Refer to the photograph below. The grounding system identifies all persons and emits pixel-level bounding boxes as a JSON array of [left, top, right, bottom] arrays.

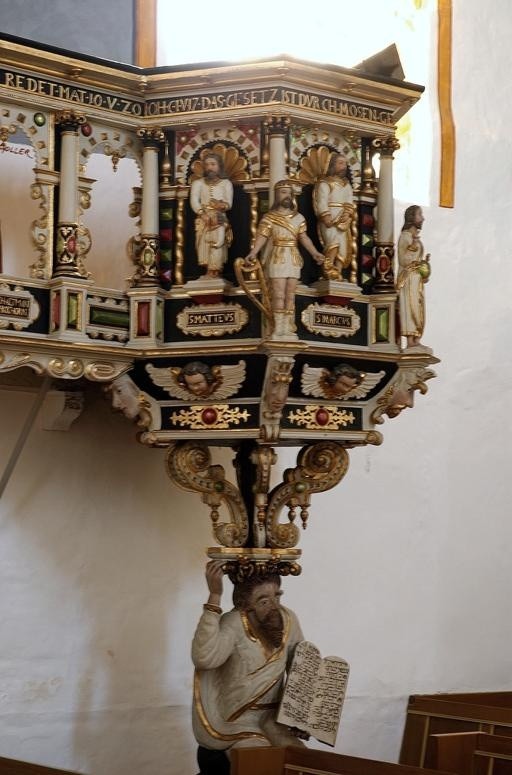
[[326, 366, 367, 396], [189, 153, 233, 274], [104, 374, 145, 421], [314, 155, 360, 278], [178, 361, 221, 396], [191, 557, 321, 753], [386, 380, 429, 419], [244, 181, 325, 340], [396, 205, 431, 349]]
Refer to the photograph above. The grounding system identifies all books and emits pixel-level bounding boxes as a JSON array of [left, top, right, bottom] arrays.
[[276, 641, 349, 747]]
